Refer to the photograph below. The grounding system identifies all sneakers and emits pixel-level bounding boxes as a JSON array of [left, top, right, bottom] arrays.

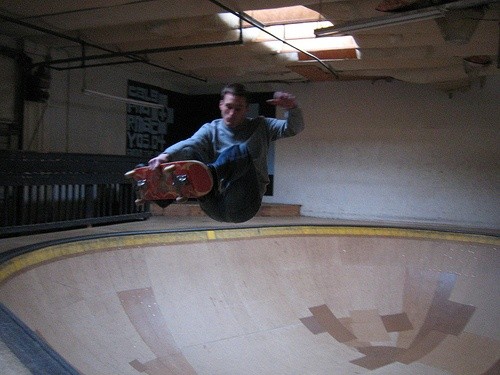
[[136, 163, 168, 208], [182, 147, 212, 172]]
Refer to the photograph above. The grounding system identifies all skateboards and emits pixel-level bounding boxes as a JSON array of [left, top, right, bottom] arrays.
[[125, 160, 212, 205]]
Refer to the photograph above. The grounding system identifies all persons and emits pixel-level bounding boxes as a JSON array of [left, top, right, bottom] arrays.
[[136, 82, 305, 223]]
